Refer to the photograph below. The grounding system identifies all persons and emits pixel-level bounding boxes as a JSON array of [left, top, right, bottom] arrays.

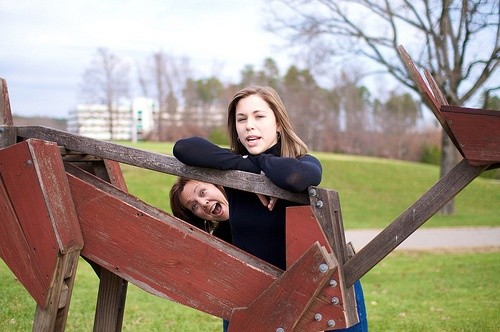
[[172, 86, 368, 332], [169, 176, 232, 244]]
